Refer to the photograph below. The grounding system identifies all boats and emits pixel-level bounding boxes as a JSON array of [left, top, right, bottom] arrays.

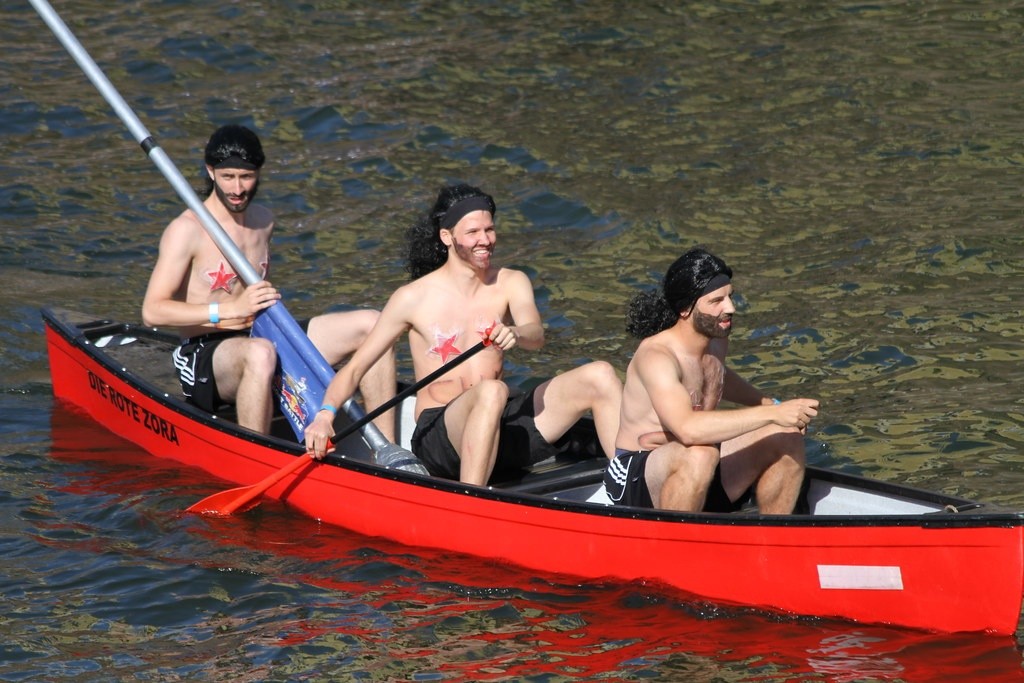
[[45, 309, 1022, 638]]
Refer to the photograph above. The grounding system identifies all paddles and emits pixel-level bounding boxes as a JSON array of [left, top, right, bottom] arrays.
[[187, 322, 503, 517]]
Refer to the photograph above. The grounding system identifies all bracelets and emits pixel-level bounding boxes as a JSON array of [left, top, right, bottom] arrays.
[[209, 302, 218, 324], [318, 405, 336, 416], [772, 398, 781, 405]]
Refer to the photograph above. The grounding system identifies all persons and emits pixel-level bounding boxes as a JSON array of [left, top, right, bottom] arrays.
[[603, 247, 820, 515], [141, 125, 397, 442], [305, 185, 624, 485]]
